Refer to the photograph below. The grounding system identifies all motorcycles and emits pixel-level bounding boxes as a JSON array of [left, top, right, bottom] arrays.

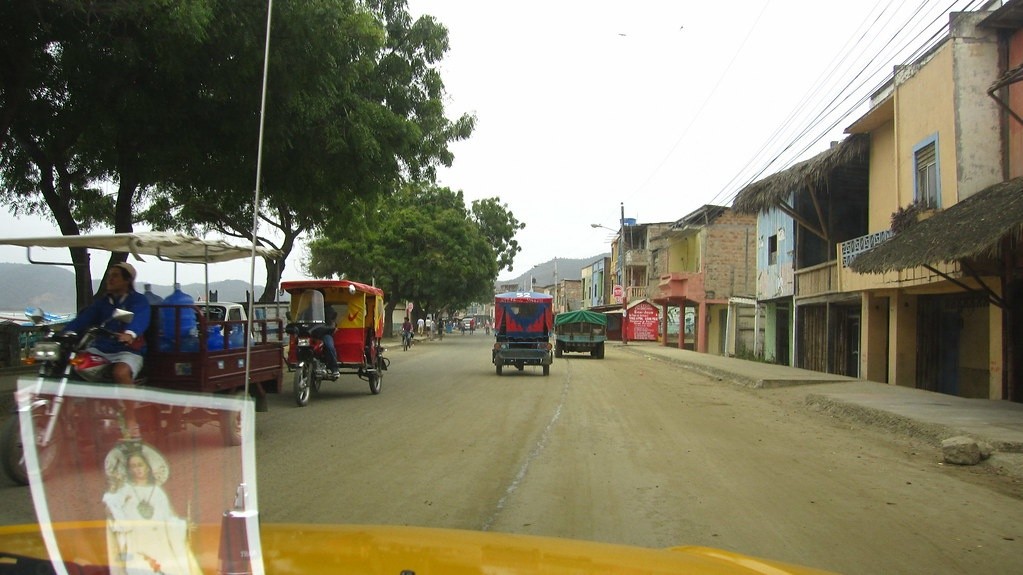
[[490, 290, 555, 377], [280, 280, 389, 408], [2, 232, 285, 483]]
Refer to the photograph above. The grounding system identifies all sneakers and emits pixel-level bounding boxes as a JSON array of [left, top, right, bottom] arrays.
[[332, 371, 341, 378]]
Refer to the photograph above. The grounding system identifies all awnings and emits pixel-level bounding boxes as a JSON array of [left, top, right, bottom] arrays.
[[851, 176, 1021, 318], [729, 131, 867, 243]]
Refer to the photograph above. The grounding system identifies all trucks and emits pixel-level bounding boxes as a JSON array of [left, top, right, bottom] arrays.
[[555, 310, 607, 359]]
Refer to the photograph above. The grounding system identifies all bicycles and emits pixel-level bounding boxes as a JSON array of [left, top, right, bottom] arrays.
[[401, 331, 416, 351]]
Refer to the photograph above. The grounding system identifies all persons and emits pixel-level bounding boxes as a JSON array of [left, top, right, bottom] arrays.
[[417, 315, 496, 342], [400, 316, 413, 348], [296, 288, 341, 378], [55, 261, 154, 428]]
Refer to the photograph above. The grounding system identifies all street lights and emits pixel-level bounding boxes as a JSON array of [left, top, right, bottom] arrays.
[[591, 202, 628, 345]]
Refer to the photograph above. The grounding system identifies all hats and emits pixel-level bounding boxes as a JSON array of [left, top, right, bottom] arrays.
[[110, 261, 136, 281]]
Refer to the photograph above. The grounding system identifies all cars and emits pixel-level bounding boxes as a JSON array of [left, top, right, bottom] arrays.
[[444, 315, 491, 334]]
